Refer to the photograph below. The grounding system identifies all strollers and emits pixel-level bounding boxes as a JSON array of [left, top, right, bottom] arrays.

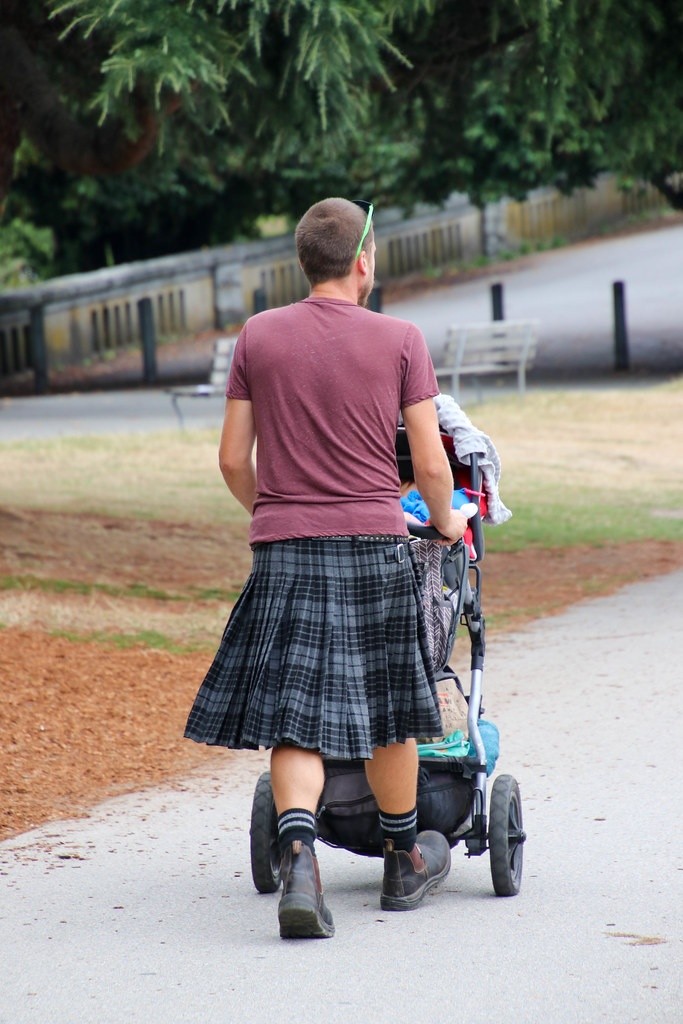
[[250, 394, 529, 897]]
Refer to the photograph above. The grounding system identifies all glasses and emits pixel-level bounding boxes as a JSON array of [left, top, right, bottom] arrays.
[[351, 200, 375, 259]]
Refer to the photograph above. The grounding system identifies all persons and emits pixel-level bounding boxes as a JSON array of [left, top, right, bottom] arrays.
[[395, 429, 472, 527], [184, 198, 467, 938]]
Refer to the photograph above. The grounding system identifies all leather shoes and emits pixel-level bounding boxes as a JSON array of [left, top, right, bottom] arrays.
[[278, 840, 335, 938], [380, 831, 451, 911]]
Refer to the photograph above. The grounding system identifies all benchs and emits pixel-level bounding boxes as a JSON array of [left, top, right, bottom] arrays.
[[164, 336, 236, 431], [434, 319, 538, 401]]
